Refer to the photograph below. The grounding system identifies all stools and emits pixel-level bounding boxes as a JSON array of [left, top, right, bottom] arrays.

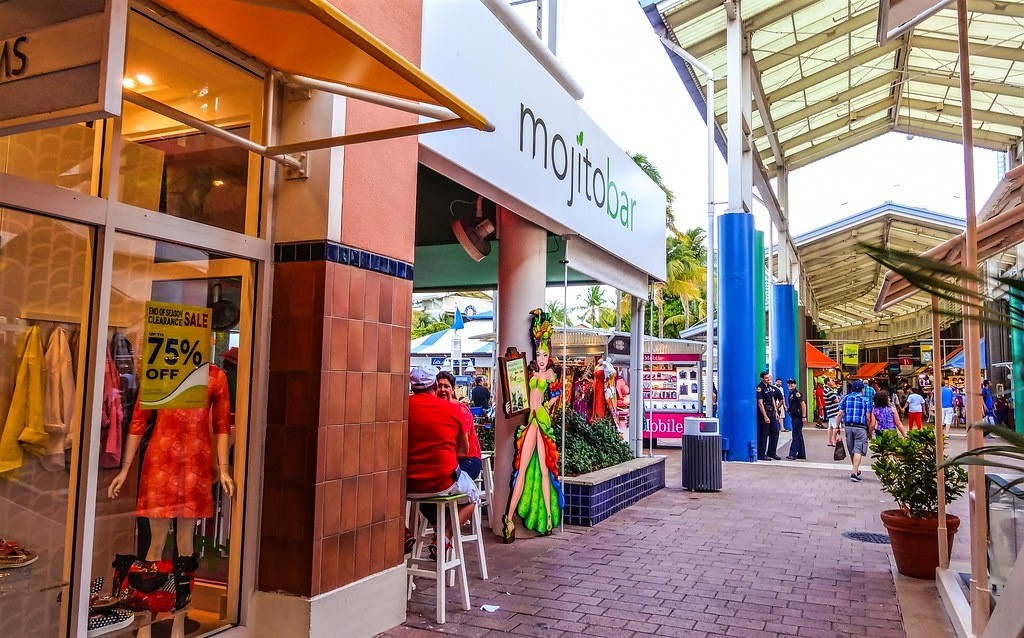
[[406, 449, 493, 624]]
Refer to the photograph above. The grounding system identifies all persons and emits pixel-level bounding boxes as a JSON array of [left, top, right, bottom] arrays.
[[403, 364, 492, 560], [758, 369, 1013, 484], [110, 338, 232, 638]]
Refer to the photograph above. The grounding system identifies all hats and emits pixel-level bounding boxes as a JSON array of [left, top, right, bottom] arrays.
[[851, 379, 866, 389], [409, 363, 440, 390], [218, 346, 238, 366], [785, 378, 796, 383]]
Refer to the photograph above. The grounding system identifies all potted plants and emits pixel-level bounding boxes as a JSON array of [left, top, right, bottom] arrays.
[[868, 425, 968, 580]]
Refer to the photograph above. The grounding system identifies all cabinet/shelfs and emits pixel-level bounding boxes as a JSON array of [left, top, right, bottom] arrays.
[[643, 369, 677, 399]]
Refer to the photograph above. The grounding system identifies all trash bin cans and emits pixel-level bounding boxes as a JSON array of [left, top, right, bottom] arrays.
[[681, 415, 723, 491]]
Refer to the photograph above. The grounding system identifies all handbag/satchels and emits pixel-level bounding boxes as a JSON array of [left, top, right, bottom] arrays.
[[833, 435, 846, 461]]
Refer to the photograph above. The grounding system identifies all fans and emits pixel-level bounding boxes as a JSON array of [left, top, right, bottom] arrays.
[[452, 194, 495, 263]]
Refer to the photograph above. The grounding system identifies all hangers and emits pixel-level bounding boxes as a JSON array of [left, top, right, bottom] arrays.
[[580, 360, 594, 382]]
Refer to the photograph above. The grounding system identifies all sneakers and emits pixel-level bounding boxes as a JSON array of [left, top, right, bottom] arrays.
[[0, 538, 40, 570]]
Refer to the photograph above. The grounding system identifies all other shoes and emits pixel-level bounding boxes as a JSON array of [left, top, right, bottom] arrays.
[[850, 470, 862, 482], [405, 526, 416, 551], [786, 455, 797, 460], [781, 429, 789, 432], [827, 443, 836, 447], [946, 435, 952, 440], [985, 434, 995, 440], [816, 422, 827, 429], [767, 454, 781, 460], [796, 454, 806, 459], [58, 576, 135, 638], [757, 454, 773, 460], [427, 539, 454, 563]]
